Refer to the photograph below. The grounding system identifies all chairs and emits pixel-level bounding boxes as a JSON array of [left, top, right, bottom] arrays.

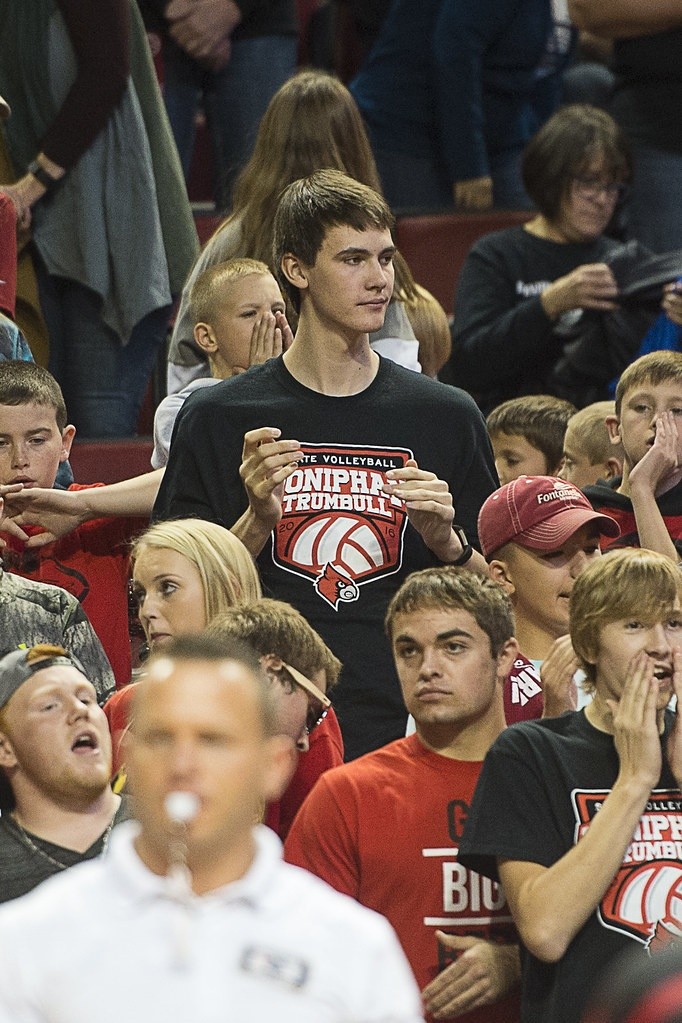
[[60, 439, 157, 553], [397, 209, 534, 310]]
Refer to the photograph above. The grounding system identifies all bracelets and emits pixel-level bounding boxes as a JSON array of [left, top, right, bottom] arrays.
[[28, 161, 63, 194]]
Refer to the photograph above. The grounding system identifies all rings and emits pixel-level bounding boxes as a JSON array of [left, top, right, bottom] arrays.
[[202, 46, 211, 56]]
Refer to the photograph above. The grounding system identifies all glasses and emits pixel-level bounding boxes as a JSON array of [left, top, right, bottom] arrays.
[[571, 176, 624, 201], [281, 663, 331, 731]]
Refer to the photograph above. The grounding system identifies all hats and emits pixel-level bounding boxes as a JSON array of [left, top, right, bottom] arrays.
[[477, 474, 622, 558], [0, 647, 86, 708]]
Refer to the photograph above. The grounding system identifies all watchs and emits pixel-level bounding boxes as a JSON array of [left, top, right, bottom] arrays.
[[430, 525, 472, 567]]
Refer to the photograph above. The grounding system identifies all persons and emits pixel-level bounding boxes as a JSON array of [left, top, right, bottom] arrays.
[[0, 360, 165, 708], [0, 643, 131, 905], [0, 311, 74, 489], [149, 75, 454, 471], [136, 0, 682, 272], [148, 167, 501, 753], [1, 0, 197, 434], [0, 631, 426, 1023], [285, 348, 682, 1022], [96, 516, 343, 838], [454, 100, 681, 395]]
